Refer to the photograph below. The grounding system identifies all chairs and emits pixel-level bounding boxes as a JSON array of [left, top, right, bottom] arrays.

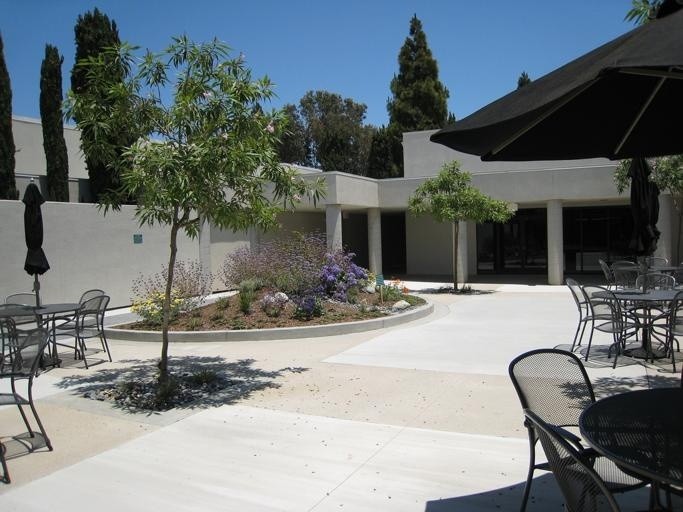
[[1, 289, 112, 486], [565, 255, 682, 381], [522, 409, 666, 512], [509, 351, 663, 512]]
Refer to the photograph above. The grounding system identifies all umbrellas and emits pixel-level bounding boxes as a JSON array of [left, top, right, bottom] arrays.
[[622, 159, 668, 360], [16, 176, 64, 370], [646, 178, 662, 255], [429, 2, 683, 162]]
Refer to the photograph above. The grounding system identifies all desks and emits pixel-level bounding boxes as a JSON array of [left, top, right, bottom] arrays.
[[579, 380, 681, 512]]
